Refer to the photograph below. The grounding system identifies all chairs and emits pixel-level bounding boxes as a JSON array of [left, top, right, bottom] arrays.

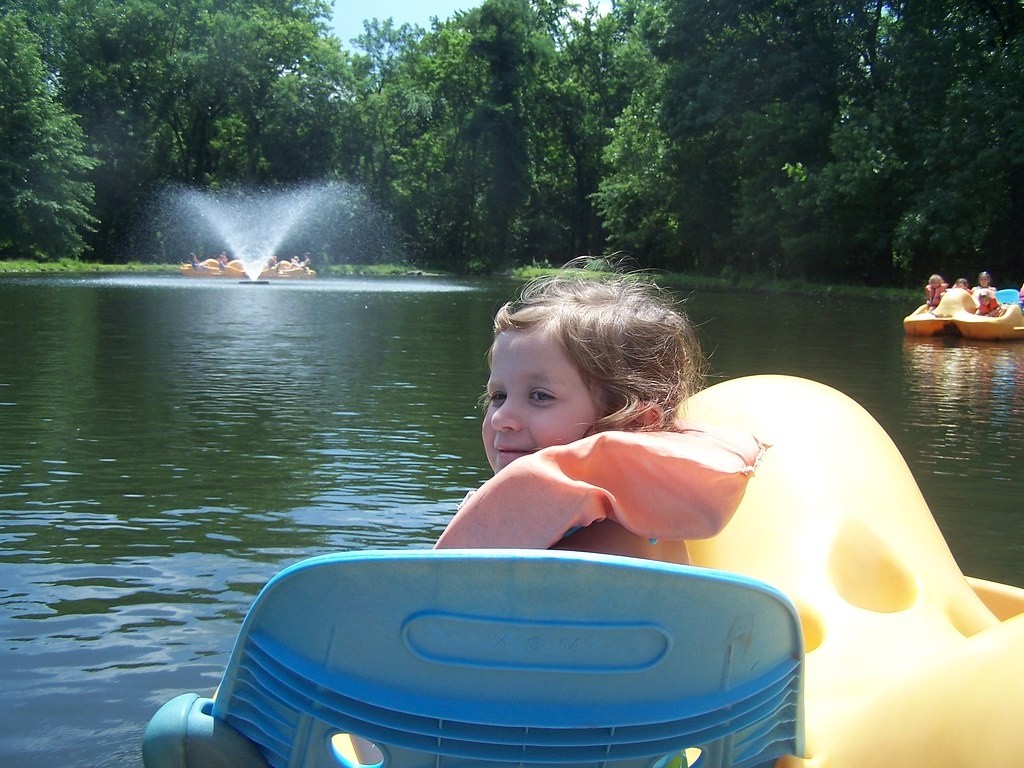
[[995, 289, 1019, 305], [138, 551, 802, 768]]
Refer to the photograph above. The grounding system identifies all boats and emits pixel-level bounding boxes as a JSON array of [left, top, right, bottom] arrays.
[[180, 258, 316, 279], [903, 288, 1024, 341]]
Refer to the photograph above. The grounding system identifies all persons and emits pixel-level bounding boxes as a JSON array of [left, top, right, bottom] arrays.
[[189, 251, 312, 271], [434, 256, 771, 565], [951, 278, 972, 295], [924, 274, 949, 310], [969, 271, 1024, 317]]
